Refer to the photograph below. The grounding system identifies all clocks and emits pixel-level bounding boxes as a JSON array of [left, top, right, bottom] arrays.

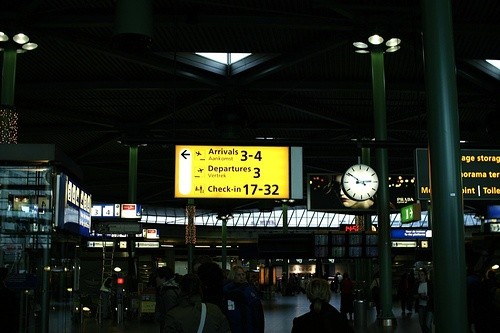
[[340, 156, 379, 201]]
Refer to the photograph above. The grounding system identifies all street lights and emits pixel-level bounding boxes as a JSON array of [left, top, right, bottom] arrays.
[[274, 199, 295, 278], [0, 30, 38, 124], [116, 140, 149, 254], [351, 32, 402, 328], [217, 213, 231, 272], [352, 138, 376, 231]]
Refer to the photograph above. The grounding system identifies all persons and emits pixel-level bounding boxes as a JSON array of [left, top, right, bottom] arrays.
[[341, 273, 354, 320], [281, 273, 300, 296], [325, 175, 374, 209], [99, 274, 117, 319], [292, 278, 352, 333], [155, 262, 264, 333], [0, 268, 8, 289], [397, 269, 432, 333]]
